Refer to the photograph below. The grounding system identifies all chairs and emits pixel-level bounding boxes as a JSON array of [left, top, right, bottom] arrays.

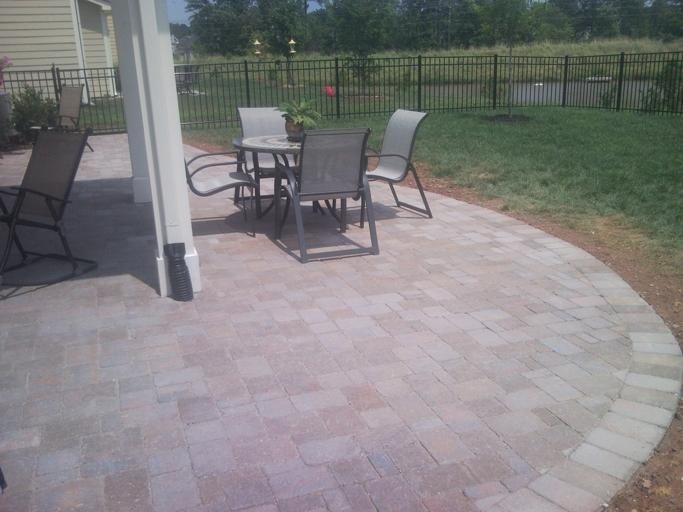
[[29, 83, 94, 152], [273, 126, 379, 263], [185, 150, 260, 229], [237, 107, 286, 182], [0, 125, 98, 286], [364, 107, 432, 218]]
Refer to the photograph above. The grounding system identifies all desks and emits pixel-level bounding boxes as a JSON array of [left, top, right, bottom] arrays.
[[232, 134, 349, 229]]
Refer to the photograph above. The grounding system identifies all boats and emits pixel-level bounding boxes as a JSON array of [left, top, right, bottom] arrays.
[[584, 73, 612, 82], [528, 81, 543, 87]]
